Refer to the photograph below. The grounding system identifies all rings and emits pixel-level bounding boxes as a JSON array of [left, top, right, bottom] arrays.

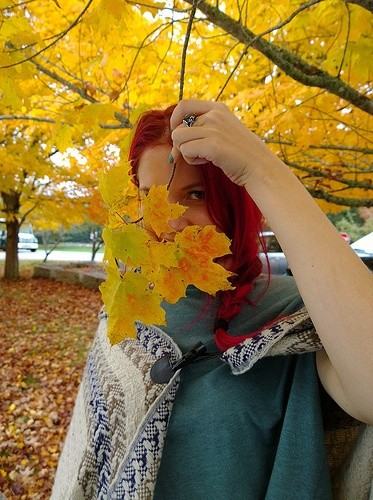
[[181, 113, 196, 129]]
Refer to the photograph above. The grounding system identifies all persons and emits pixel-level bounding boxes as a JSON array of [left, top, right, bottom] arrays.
[[48, 98, 373, 500]]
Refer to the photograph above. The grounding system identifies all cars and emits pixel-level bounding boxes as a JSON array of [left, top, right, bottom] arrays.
[[258, 228, 290, 277], [0, 218, 39, 253], [350, 231, 373, 273]]
